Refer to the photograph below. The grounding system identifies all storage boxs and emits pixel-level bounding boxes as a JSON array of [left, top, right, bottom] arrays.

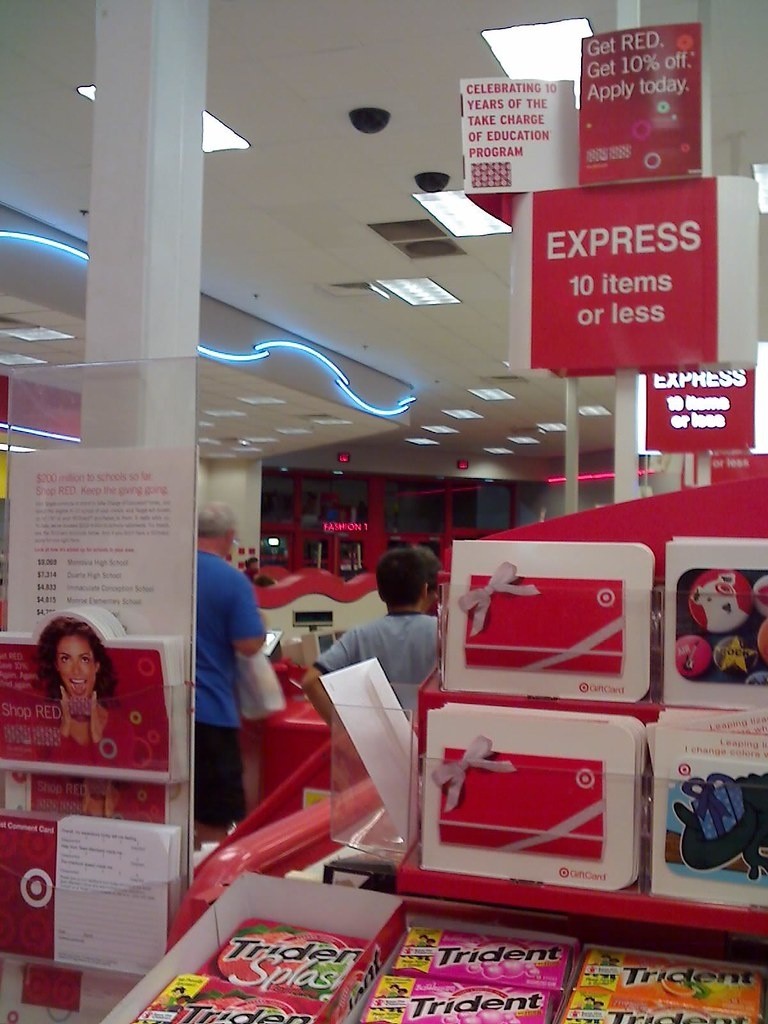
[[93, 872, 407, 1024], [555, 943, 768, 1024], [344, 916, 578, 1024]]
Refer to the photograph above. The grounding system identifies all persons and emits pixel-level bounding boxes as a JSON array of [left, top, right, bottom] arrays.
[[243, 557, 259, 583], [193, 500, 266, 850], [301, 547, 439, 731], [34, 616, 118, 744]]
